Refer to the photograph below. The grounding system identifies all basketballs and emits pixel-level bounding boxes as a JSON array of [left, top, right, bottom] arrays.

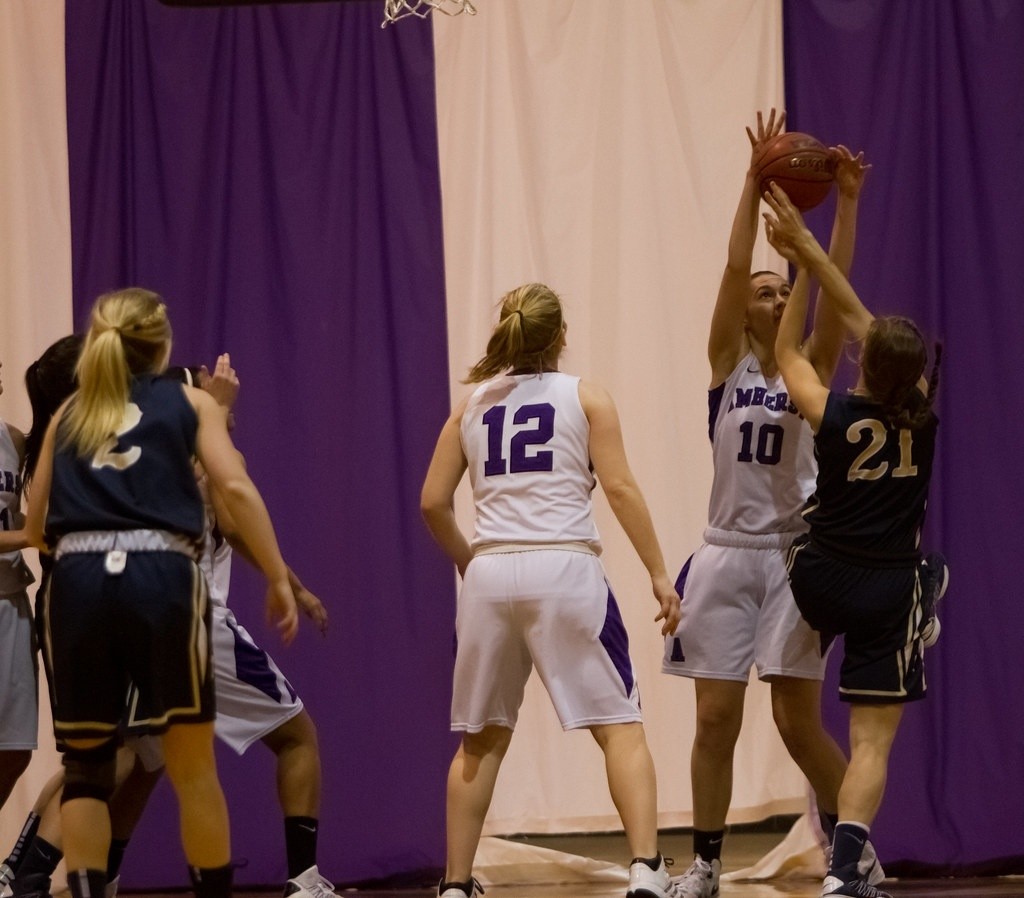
[[754, 129, 834, 213]]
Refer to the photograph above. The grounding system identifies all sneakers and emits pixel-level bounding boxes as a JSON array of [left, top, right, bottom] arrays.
[[675, 856, 720, 897], [625, 851, 687, 898], [822, 869, 895, 898], [436, 875, 485, 898], [821, 845, 886, 887], [8, 874, 52, 898], [284, 864, 343, 898], [919, 561, 952, 647]]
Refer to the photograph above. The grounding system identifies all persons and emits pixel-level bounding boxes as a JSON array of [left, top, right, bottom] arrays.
[[666, 109, 886, 897], [418, 280, 685, 896], [752, 177, 950, 898], [1, 285, 346, 897]]
